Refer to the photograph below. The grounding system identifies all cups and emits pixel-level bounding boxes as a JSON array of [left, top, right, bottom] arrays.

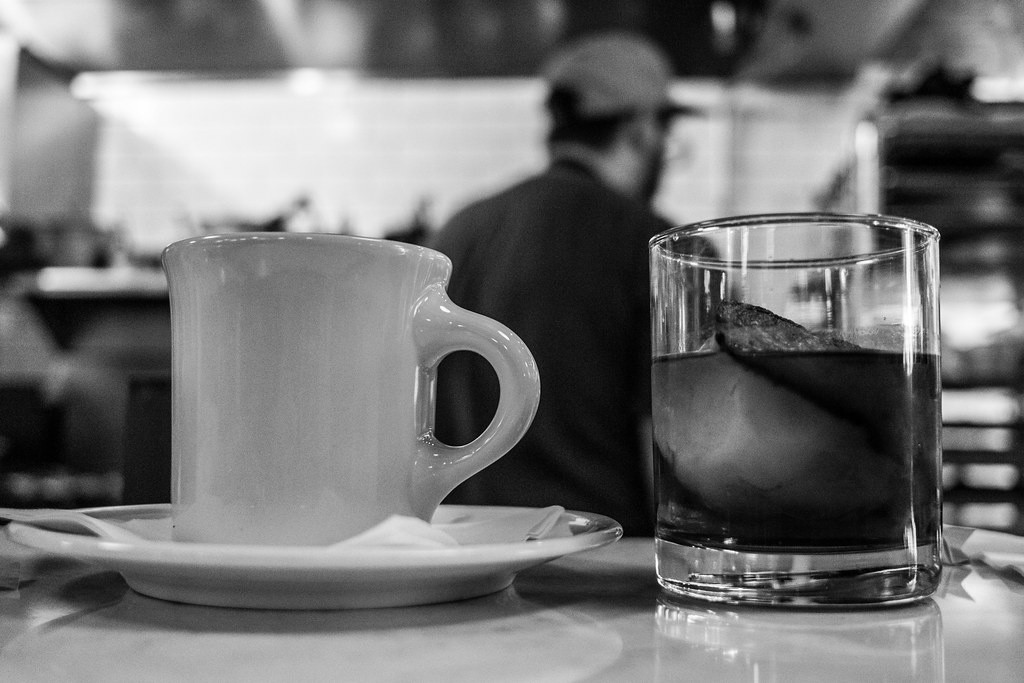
[[160, 232, 541, 546], [648, 212, 945, 605]]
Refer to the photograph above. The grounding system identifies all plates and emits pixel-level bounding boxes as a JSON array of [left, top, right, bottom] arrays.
[[3, 503, 623, 611]]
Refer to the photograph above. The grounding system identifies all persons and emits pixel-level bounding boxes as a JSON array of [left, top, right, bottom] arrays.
[[428, 30, 734, 540]]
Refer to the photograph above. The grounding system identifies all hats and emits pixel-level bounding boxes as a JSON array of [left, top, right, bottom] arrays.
[[540, 30, 708, 128]]
